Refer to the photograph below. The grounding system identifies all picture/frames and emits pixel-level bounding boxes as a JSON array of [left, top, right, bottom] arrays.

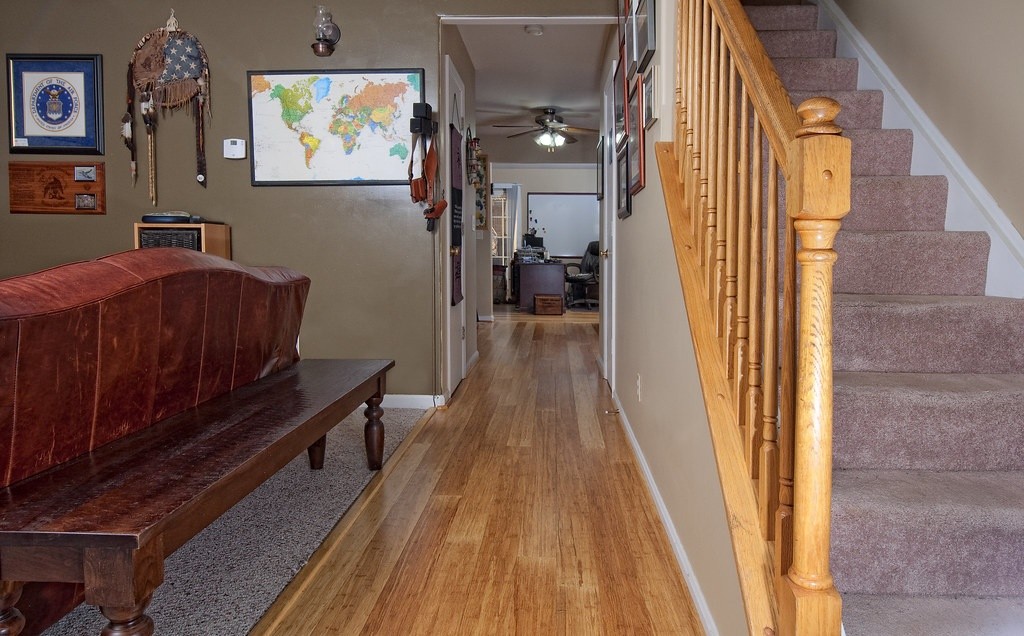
[[5, 52, 107, 157], [246, 67, 426, 188], [595, 0, 661, 220]]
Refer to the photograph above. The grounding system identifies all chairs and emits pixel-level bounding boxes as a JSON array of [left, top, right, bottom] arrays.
[[563, 241, 599, 310]]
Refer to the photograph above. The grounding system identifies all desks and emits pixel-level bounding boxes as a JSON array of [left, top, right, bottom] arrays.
[[0, 358, 398, 636], [511, 258, 567, 314]]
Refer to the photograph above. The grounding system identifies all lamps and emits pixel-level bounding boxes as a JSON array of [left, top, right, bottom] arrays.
[[310, 4, 340, 58], [533, 129, 568, 153]]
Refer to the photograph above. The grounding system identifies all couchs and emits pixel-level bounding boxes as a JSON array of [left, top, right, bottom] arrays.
[[0, 247, 313, 636]]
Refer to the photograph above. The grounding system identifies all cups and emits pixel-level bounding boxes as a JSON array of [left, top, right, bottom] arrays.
[[543, 250, 549, 262]]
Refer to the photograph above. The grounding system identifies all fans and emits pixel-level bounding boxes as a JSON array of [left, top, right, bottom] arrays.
[[491, 108, 600, 144]]
